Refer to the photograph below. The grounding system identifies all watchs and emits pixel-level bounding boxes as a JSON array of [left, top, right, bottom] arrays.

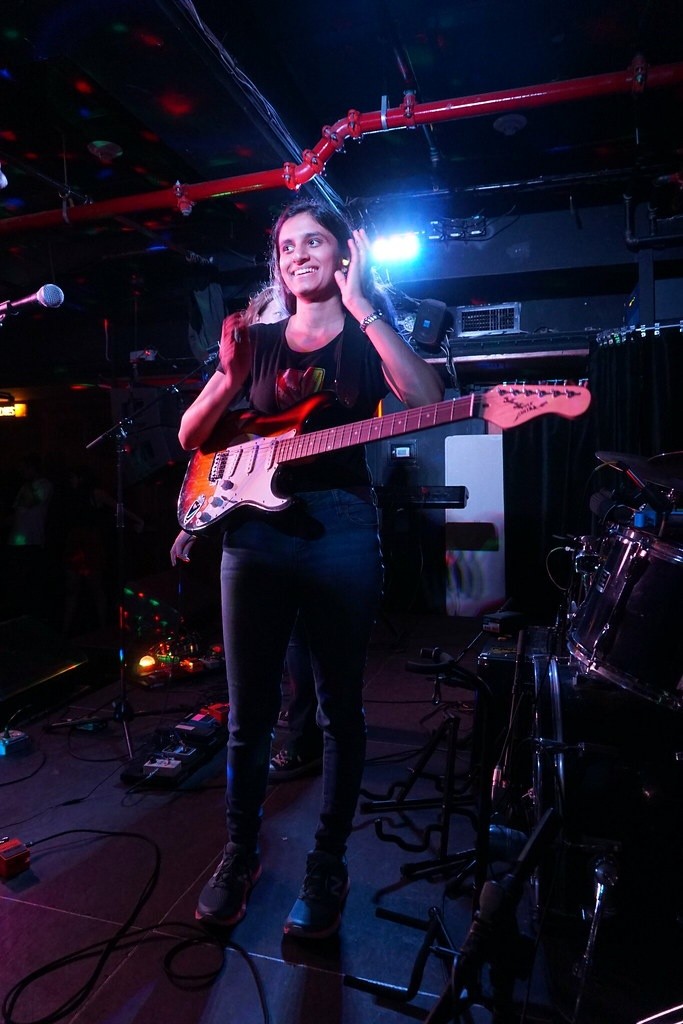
[[360, 309, 387, 333]]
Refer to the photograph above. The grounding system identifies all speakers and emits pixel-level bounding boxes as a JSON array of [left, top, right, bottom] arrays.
[[467, 625, 569, 793], [414, 299, 448, 351]]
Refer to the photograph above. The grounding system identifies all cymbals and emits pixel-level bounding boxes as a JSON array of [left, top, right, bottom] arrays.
[[590, 449, 682, 493]]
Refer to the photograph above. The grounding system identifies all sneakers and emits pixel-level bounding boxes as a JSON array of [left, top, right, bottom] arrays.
[[283, 851, 352, 940], [277, 711, 290, 727], [267, 746, 314, 780], [194, 843, 264, 927]]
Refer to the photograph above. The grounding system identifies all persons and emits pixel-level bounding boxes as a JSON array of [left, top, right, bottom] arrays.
[[170, 200, 444, 939]]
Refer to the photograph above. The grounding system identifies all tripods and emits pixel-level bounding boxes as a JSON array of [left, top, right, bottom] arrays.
[[39, 355, 216, 773]]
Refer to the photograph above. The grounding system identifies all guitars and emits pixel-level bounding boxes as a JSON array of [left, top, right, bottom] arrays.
[[173, 380, 595, 537]]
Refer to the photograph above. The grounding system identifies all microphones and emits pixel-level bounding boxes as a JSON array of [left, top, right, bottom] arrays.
[[616, 459, 673, 516], [0, 284, 64, 316]]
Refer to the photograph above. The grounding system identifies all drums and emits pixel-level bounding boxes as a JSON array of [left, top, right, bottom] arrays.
[[565, 516, 683, 711]]
